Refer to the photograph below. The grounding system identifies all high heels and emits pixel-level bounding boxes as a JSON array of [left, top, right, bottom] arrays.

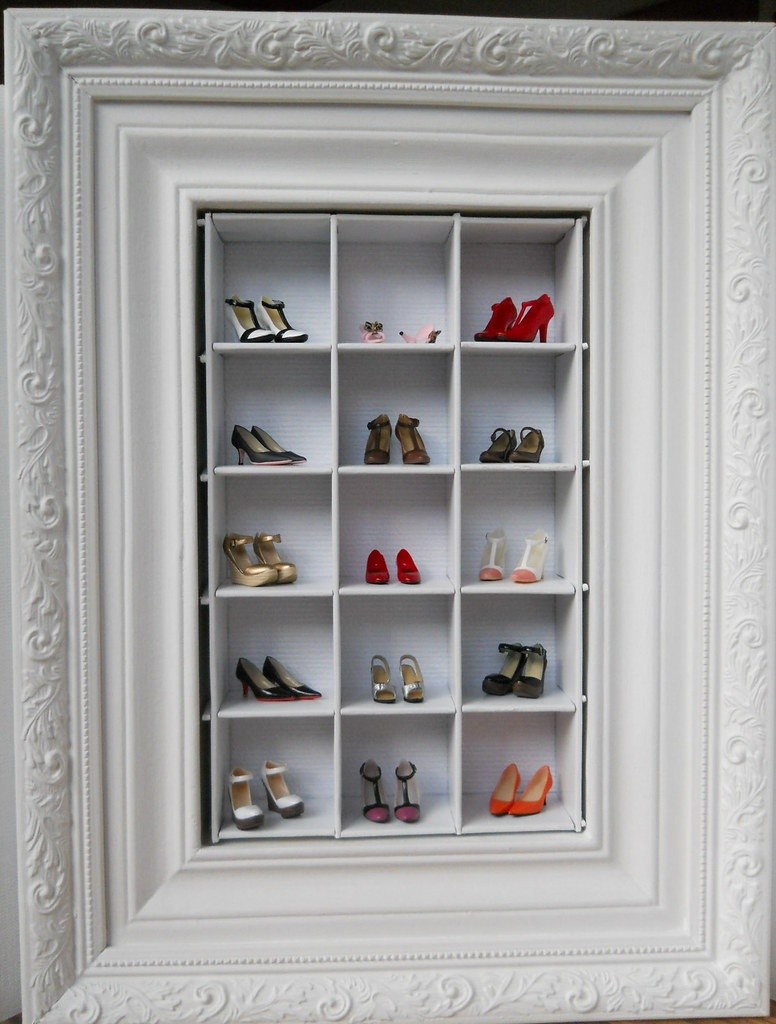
[[490, 763, 521, 816], [223, 530, 279, 587], [479, 527, 507, 581], [479, 427, 517, 463], [399, 655, 424, 703], [359, 757, 389, 823], [394, 758, 420, 821], [370, 654, 396, 703], [509, 528, 552, 583], [224, 294, 308, 343], [232, 424, 307, 465], [508, 765, 553, 816], [365, 549, 390, 584], [364, 414, 392, 464], [253, 530, 297, 584], [359, 320, 385, 342], [396, 549, 421, 584], [399, 323, 441, 344], [228, 767, 264, 830], [482, 642, 547, 699], [510, 427, 544, 463], [395, 413, 431, 464], [474, 294, 554, 343], [235, 655, 322, 701], [261, 760, 304, 819]]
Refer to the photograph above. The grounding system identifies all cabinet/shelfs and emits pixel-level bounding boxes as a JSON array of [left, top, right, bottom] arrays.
[[196, 214, 586, 844]]
